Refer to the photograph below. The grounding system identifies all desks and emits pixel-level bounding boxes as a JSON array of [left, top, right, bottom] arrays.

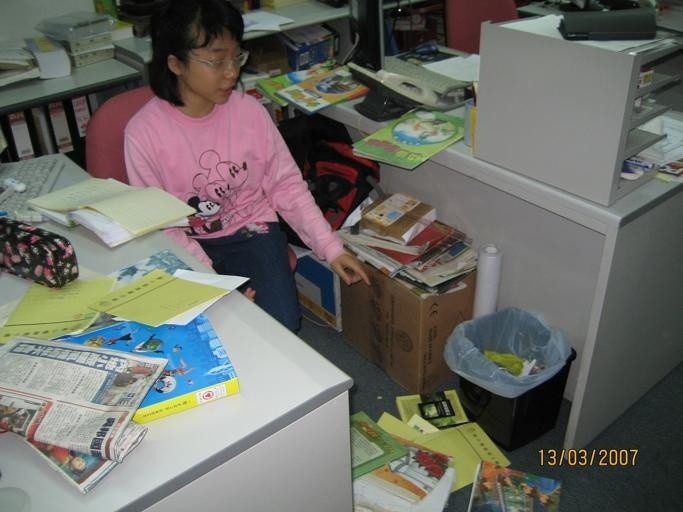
[[320, 16, 683, 459], [0, 154, 355, 512]]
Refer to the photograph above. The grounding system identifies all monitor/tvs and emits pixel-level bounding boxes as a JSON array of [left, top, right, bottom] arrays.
[[341, 0, 385, 70]]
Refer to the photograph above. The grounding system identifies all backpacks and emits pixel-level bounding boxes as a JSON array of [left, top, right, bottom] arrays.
[[277, 117, 379, 250]]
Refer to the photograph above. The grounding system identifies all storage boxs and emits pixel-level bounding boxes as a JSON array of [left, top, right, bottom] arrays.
[[340, 263, 476, 394]]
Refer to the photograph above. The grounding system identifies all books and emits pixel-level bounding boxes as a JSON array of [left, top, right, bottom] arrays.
[[335, 191, 479, 297], [0, 37, 71, 85], [348, 106, 468, 172], [28, 177, 190, 246], [274, 66, 370, 113], [48, 312, 240, 424], [255, 65, 331, 107], [25, 177, 197, 236], [349, 388, 562, 511]]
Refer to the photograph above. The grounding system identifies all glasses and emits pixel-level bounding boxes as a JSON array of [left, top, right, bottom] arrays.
[[187, 47, 250, 74]]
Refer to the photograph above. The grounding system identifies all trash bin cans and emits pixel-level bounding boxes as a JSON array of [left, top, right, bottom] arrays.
[[458, 307, 576, 451]]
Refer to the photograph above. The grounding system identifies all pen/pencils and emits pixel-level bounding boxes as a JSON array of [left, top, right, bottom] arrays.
[[471, 81, 477, 108]]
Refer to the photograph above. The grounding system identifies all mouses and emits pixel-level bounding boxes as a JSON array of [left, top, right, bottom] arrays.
[[412, 40, 438, 55]]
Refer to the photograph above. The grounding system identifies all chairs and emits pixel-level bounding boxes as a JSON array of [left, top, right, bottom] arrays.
[[85, 87, 298, 289]]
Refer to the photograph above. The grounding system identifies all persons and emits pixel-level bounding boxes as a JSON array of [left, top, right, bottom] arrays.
[[25, 438, 88, 476], [121, 2, 370, 333]]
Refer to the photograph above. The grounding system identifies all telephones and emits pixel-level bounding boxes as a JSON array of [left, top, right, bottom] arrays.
[[345, 61, 438, 122]]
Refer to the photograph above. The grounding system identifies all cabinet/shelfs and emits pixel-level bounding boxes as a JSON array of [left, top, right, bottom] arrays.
[[0, 55, 141, 170], [112, 3, 351, 171]]
